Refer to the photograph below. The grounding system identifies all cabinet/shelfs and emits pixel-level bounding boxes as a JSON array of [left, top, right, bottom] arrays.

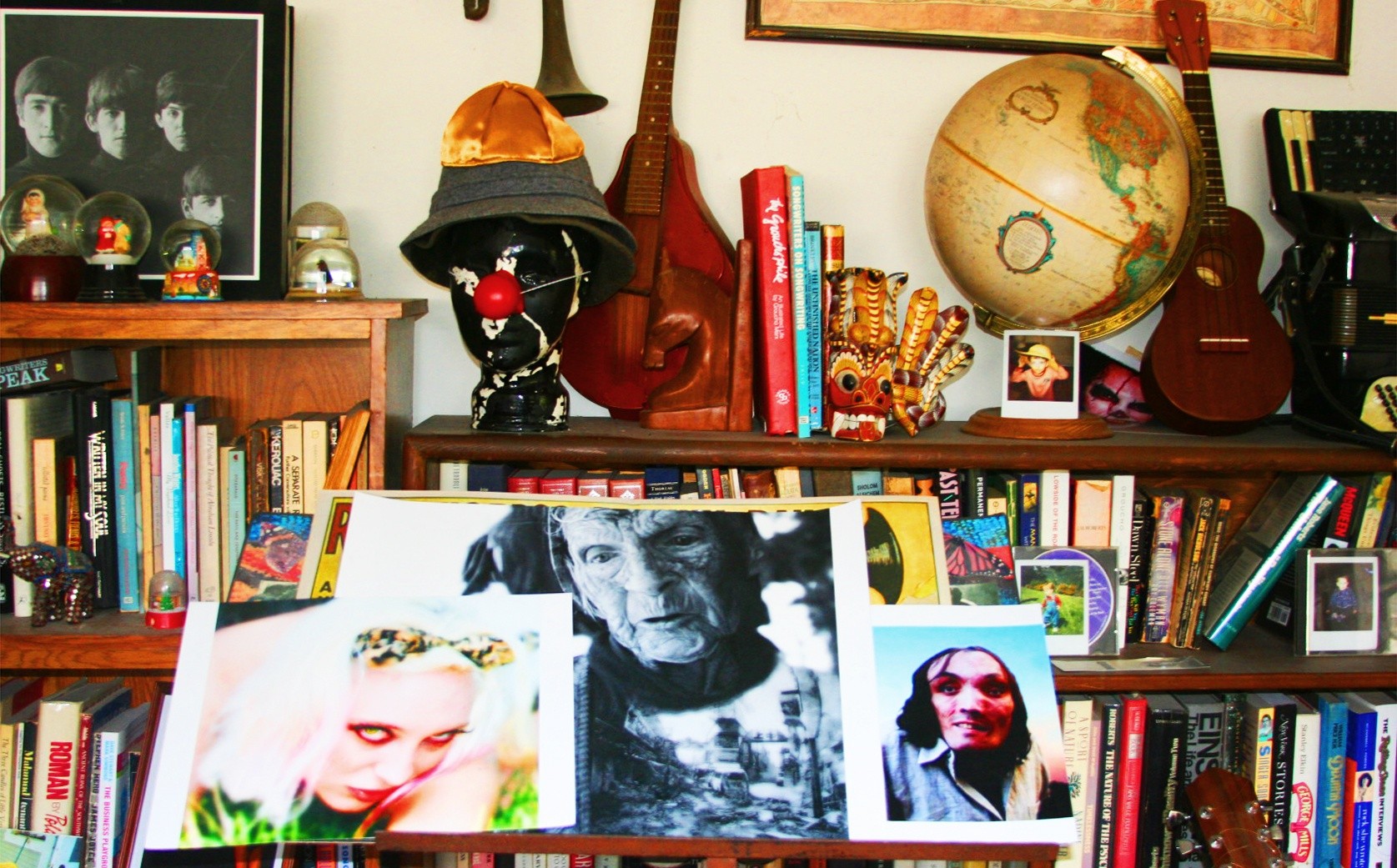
[[1, 292, 429, 867], [409, 415, 1396, 867]]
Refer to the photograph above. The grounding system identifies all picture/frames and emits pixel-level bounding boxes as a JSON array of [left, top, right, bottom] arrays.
[[743, 0, 1354, 77], [1294, 548, 1396, 657]]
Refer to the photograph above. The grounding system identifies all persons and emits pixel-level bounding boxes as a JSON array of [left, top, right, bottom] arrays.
[[178, 154, 250, 275], [146, 67, 231, 178], [444, 209, 595, 433], [80, 61, 175, 224], [7, 51, 110, 195]]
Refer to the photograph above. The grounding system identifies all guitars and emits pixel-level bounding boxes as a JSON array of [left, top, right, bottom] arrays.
[[556, 0, 739, 415], [1139, 1, 1295, 429], [1164, 764, 1291, 868]]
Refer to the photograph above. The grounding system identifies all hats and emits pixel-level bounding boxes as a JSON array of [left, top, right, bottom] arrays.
[[410, 211, 636, 310], [400, 81, 640, 289]]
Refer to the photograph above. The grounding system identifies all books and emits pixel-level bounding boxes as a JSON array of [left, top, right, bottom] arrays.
[[0, 344, 1394, 868]]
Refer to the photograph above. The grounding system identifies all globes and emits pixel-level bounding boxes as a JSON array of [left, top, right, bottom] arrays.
[[923, 43, 1208, 442]]
